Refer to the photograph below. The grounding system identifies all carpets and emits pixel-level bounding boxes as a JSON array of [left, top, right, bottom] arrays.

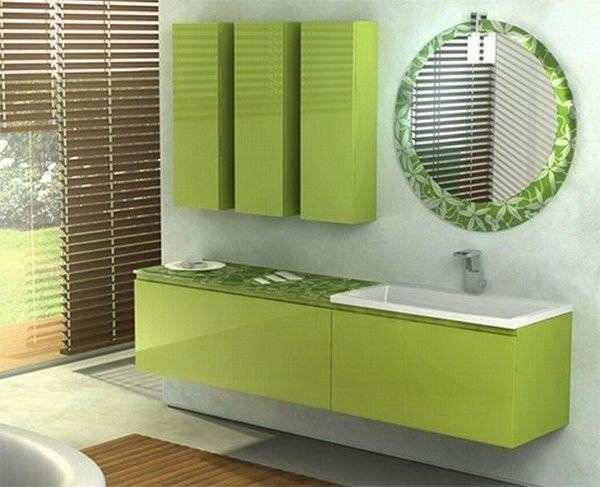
[[78, 433, 344, 487]]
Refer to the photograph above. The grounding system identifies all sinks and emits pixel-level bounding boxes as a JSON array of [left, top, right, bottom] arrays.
[[331, 281, 572, 327], [0, 425, 107, 487]]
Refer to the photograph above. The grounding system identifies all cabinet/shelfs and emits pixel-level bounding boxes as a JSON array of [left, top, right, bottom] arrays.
[[133, 279, 574, 450], [233, 23, 300, 218], [172, 22, 235, 212], [299, 21, 378, 224]]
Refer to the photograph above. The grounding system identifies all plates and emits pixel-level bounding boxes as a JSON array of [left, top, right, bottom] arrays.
[[165, 260, 226, 273]]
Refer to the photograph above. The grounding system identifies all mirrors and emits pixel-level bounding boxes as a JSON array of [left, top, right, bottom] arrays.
[[392, 19, 577, 232]]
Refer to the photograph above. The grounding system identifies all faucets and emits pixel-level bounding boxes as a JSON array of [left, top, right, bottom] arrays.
[[452, 249, 488, 295]]
